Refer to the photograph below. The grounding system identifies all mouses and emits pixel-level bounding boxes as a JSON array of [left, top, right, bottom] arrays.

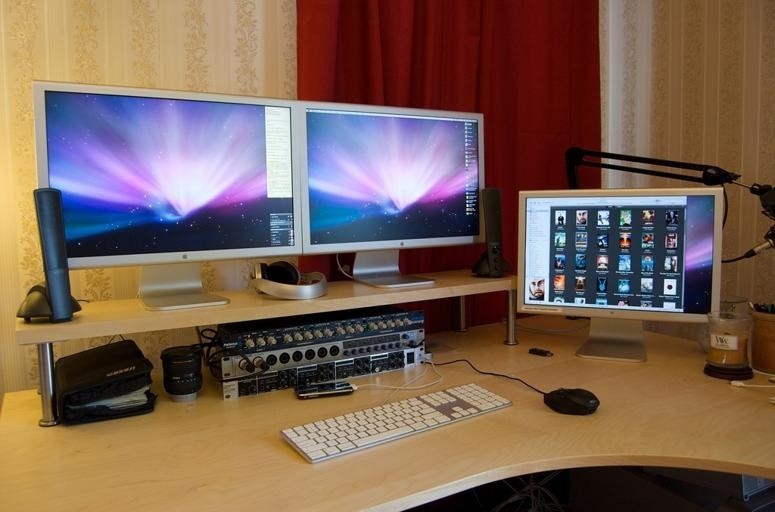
[[544, 389, 601, 415]]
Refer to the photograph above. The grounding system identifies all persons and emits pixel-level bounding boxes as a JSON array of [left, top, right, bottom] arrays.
[[576, 210, 587, 225], [554, 213, 565, 226], [528, 279, 544, 298]]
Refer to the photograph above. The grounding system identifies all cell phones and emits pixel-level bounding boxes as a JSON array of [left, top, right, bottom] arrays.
[[295, 382, 354, 398]]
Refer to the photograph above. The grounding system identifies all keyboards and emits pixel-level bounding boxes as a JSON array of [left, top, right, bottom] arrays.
[[280, 381, 511, 466]]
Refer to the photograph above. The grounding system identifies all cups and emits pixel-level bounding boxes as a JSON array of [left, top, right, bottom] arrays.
[[160, 345, 204, 394], [705, 310, 754, 370]]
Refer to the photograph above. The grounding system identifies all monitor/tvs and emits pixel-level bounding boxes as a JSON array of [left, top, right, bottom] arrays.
[[297, 99, 487, 290], [32, 79, 297, 312], [516, 188, 721, 364]]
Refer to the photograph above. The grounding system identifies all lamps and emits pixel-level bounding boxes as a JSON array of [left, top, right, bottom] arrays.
[[565, 146, 774, 262]]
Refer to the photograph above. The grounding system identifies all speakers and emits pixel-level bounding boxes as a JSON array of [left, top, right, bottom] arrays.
[[482, 189, 503, 276], [34, 187, 71, 324]]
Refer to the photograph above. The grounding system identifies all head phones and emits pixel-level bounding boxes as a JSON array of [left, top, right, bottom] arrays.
[[249, 261, 329, 299]]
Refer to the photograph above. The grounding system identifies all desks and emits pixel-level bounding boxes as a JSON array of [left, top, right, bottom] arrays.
[[2, 314, 775, 510]]
